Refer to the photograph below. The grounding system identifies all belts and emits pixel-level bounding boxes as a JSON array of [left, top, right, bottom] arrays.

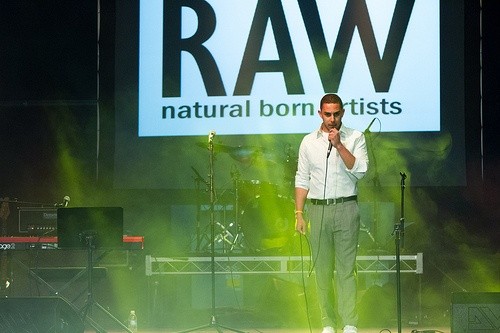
[[311, 196, 356, 205]]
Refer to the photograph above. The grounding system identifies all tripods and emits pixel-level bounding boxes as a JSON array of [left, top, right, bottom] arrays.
[[57, 206, 133, 333], [176, 143, 248, 333]]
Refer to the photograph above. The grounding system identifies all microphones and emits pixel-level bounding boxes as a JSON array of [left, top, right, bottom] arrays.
[[61, 195, 70, 207], [364, 118, 376, 135], [327, 142, 333, 158], [209, 131, 216, 143]]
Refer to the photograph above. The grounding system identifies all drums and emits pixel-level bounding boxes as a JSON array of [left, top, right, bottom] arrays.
[[235, 179, 274, 214], [284, 156, 299, 188], [240, 194, 297, 252]]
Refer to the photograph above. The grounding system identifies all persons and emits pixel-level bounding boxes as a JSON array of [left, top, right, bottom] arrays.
[[294, 94, 369, 333]]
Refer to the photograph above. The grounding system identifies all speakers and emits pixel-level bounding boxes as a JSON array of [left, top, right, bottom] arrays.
[[0, 294, 85, 333], [450, 292, 500, 333]]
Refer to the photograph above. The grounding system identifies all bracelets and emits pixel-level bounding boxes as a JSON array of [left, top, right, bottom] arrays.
[[295, 211, 303, 214]]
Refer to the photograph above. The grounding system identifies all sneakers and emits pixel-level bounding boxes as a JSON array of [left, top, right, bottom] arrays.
[[343, 326, 357, 333], [323, 327, 334, 333]]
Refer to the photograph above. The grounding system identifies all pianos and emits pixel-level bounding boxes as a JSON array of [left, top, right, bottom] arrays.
[[0, 234, 143, 333]]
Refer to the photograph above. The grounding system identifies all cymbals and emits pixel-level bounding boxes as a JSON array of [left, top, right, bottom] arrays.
[[209, 186, 234, 190], [196, 142, 234, 152], [229, 146, 282, 165]]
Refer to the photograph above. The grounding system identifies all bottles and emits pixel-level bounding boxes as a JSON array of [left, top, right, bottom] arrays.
[[128, 310, 137, 333]]
